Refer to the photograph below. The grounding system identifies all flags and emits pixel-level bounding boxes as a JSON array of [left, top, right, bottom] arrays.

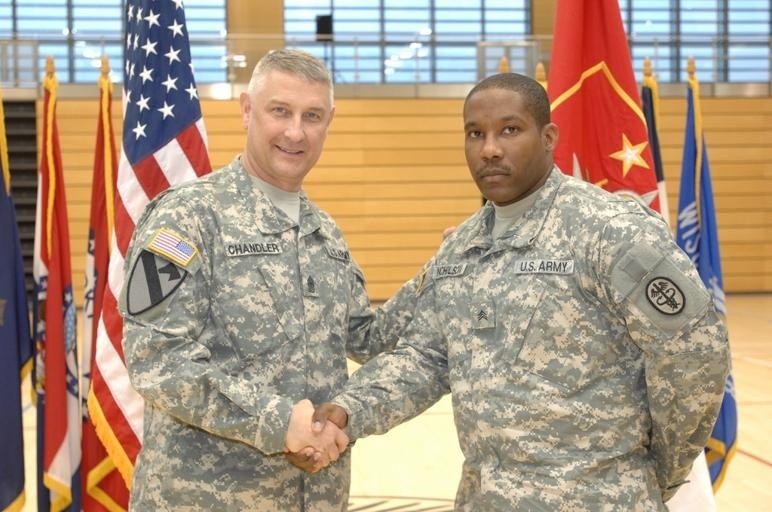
[[37, 53, 85, 511], [675, 56, 737, 493], [82, 1, 215, 510], [640, 58, 670, 229], [1, 90, 37, 512], [544, 1, 659, 216], [86, 57, 121, 399]]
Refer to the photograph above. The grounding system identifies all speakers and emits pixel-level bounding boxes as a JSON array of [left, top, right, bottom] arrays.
[[315, 14, 332, 41]]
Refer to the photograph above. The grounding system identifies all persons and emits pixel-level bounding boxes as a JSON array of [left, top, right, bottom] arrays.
[[286, 72, 733, 511], [116, 46, 459, 511]]
